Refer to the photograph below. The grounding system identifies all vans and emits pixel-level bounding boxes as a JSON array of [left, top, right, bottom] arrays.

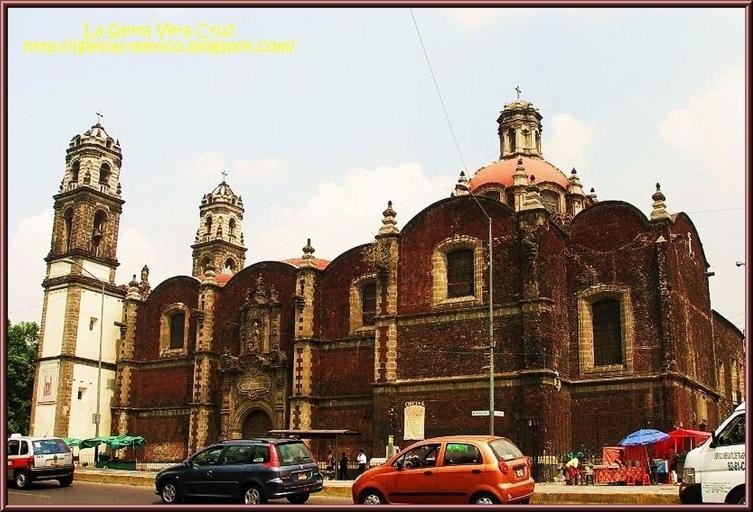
[[679, 401, 748, 504]]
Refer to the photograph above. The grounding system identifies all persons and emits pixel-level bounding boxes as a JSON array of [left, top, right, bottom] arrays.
[[565, 451, 584, 485], [396, 449, 419, 463], [326, 452, 351, 480], [356, 449, 367, 475]]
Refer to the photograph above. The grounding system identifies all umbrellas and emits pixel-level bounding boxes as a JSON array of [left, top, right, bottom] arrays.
[[617, 429, 671, 474]]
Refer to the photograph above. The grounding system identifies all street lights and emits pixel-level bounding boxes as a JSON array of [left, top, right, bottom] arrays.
[[455, 182, 494, 435], [59, 258, 106, 462]]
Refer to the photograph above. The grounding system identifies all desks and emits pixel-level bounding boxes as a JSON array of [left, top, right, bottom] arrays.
[[580, 466, 642, 486]]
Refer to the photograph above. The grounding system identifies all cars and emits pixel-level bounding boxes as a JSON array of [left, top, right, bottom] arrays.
[[352, 434, 535, 505], [155, 436, 324, 504], [7, 435, 75, 490]]
